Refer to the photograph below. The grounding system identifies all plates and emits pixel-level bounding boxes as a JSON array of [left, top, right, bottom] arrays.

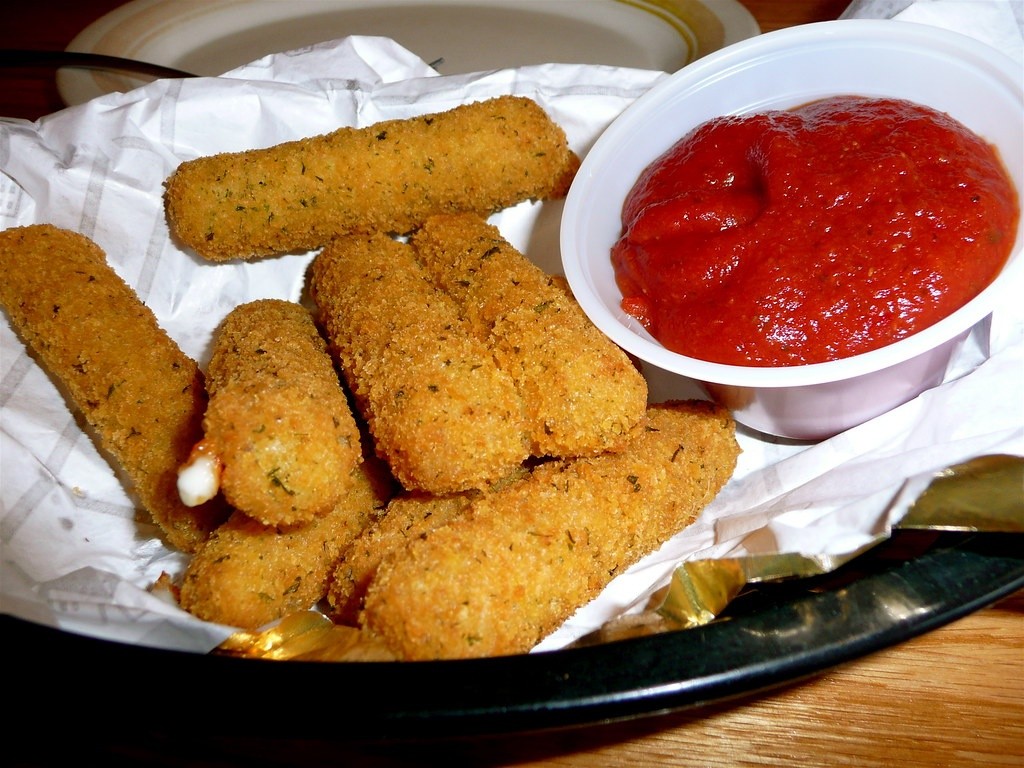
[[55, 0, 765, 109], [0, 535, 1024, 746]]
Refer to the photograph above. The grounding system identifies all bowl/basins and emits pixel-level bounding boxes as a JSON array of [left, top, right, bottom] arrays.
[[560, 17, 1024, 441]]
